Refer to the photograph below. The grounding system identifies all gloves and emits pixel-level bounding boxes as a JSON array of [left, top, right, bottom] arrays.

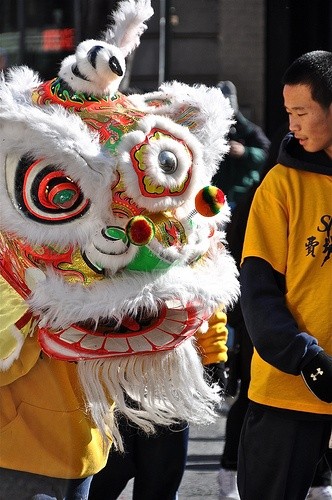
[[301, 348, 332, 407]]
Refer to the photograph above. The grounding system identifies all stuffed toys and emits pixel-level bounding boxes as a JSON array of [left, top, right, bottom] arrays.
[[2, 0, 245, 455]]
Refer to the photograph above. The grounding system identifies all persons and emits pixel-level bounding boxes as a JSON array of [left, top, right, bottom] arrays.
[[212, 317, 331, 500], [235, 48, 332, 500], [214, 79, 270, 402], [88, 303, 232, 500], [1, 324, 125, 500]]
